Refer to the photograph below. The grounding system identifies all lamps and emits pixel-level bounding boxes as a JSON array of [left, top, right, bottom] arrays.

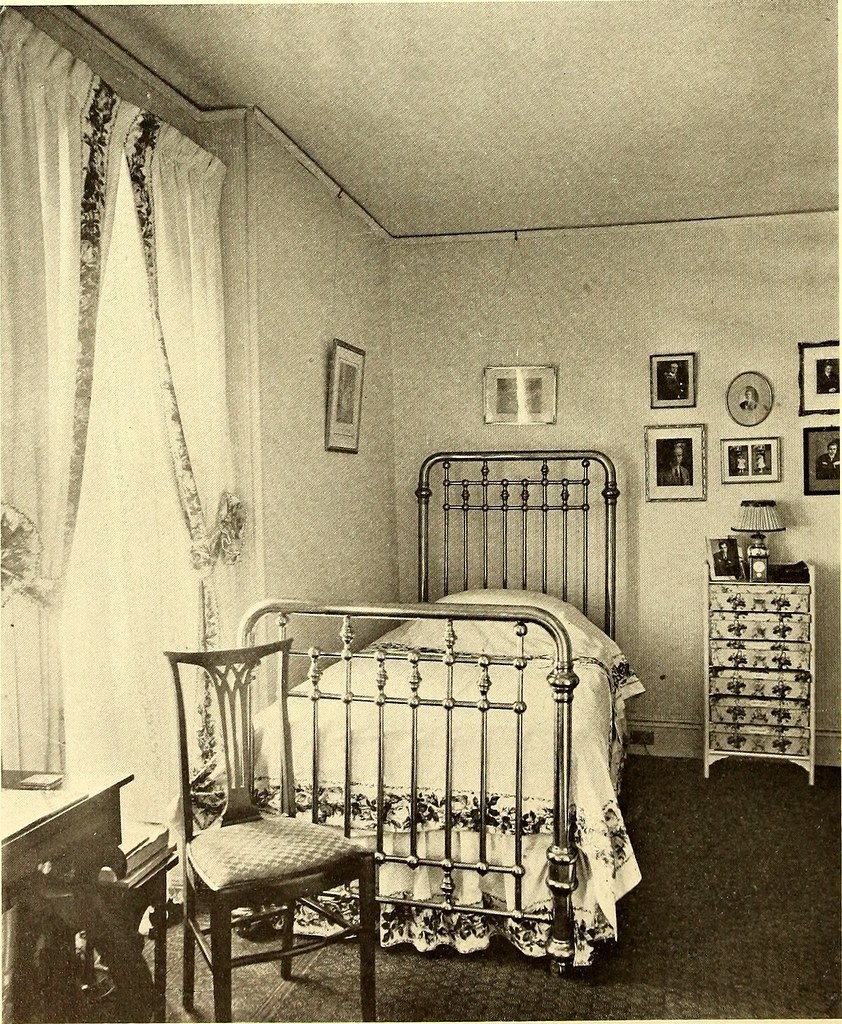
[[731, 500, 786, 583]]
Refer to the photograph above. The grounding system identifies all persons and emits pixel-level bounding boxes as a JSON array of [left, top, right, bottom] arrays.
[[739, 390, 757, 410], [819, 364, 840, 392], [737, 456, 746, 473], [817, 442, 840, 479], [658, 363, 684, 398], [658, 441, 690, 485], [713, 542, 734, 576], [755, 454, 765, 472]]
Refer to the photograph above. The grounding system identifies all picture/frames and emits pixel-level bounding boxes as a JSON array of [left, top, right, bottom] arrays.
[[649, 352, 696, 409], [803, 426, 840, 496], [705, 536, 748, 582], [798, 340, 840, 417], [483, 365, 558, 425], [720, 436, 781, 484], [643, 424, 708, 503], [325, 338, 365, 454], [724, 371, 773, 427]]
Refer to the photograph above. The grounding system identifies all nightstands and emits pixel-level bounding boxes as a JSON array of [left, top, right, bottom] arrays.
[[702, 560, 816, 786]]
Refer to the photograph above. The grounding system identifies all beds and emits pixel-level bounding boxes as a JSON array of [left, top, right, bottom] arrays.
[[169, 451, 647, 978]]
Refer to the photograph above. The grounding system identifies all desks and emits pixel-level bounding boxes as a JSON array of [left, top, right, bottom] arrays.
[[62, 854, 180, 1023], [1, 772, 154, 1024]]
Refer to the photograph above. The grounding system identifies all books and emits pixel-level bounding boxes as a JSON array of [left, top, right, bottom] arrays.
[[119, 820, 177, 888], [20, 774, 63, 789]]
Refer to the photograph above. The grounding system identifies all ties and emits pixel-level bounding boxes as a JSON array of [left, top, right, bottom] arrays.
[[745, 401, 749, 406], [675, 468, 679, 477]]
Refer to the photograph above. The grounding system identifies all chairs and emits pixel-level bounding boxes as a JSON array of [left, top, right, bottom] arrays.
[[161, 637, 378, 1023]]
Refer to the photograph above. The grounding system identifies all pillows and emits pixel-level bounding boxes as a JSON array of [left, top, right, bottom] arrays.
[[386, 589, 592, 660]]
